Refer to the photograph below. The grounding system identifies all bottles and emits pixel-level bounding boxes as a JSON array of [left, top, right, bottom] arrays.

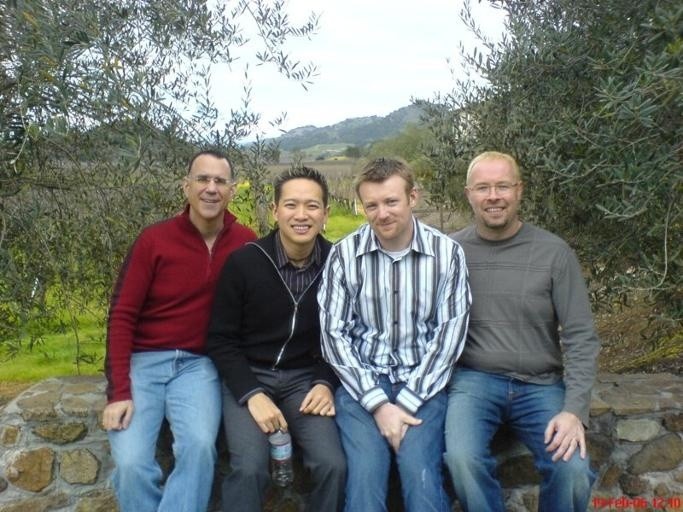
[[268, 429, 294, 486]]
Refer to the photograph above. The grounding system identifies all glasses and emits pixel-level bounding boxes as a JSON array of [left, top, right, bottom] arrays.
[[182, 173, 232, 185], [464, 180, 518, 194]]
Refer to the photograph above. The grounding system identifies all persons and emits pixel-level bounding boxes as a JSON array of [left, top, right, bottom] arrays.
[[439, 151, 601, 512], [200, 164, 349, 511], [100, 149, 259, 512], [314, 153, 472, 511]]
